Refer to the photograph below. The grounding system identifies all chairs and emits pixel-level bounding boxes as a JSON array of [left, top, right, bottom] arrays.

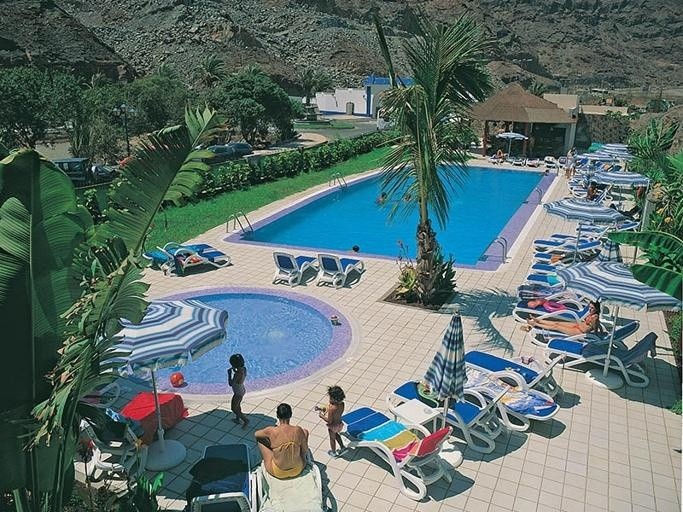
[[529, 320, 640, 347], [489, 148, 568, 167], [141, 243, 233, 276], [567, 158, 622, 200], [544, 331, 659, 390], [517, 219, 641, 299], [332, 349, 570, 505], [511, 300, 593, 325], [189, 444, 271, 511], [72, 402, 150, 486], [270, 249, 367, 289]]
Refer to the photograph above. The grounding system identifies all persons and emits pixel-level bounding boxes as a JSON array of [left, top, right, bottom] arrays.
[[401, 194, 411, 203], [317, 384, 346, 457], [586, 180, 599, 201], [495, 146, 505, 160], [227, 354, 250, 429], [570, 146, 577, 175], [349, 245, 359, 254], [565, 149, 572, 178], [526, 298, 601, 338], [634, 185, 646, 200], [374, 192, 387, 204], [254, 402, 309, 480]]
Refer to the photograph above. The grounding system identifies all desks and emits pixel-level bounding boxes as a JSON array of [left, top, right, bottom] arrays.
[[122, 391, 189, 442]]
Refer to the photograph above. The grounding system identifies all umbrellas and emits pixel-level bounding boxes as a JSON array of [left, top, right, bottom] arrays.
[[595, 238, 624, 264], [578, 144, 636, 169], [589, 170, 650, 207], [553, 259, 682, 360], [92, 297, 230, 430], [540, 195, 631, 262], [494, 132, 528, 156], [422, 309, 469, 427]]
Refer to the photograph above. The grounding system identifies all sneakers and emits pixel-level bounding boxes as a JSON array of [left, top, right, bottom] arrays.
[[328, 447, 348, 459]]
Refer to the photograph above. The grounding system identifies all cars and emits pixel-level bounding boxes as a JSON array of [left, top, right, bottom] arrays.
[[89, 140, 252, 183]]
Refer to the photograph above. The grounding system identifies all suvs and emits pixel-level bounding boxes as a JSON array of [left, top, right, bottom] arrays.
[[50, 156, 101, 186]]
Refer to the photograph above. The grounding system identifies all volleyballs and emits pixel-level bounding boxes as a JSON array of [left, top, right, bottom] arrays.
[[170, 373, 184, 386]]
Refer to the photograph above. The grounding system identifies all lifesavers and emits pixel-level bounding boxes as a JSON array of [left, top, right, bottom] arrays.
[[543, 301, 567, 312]]
